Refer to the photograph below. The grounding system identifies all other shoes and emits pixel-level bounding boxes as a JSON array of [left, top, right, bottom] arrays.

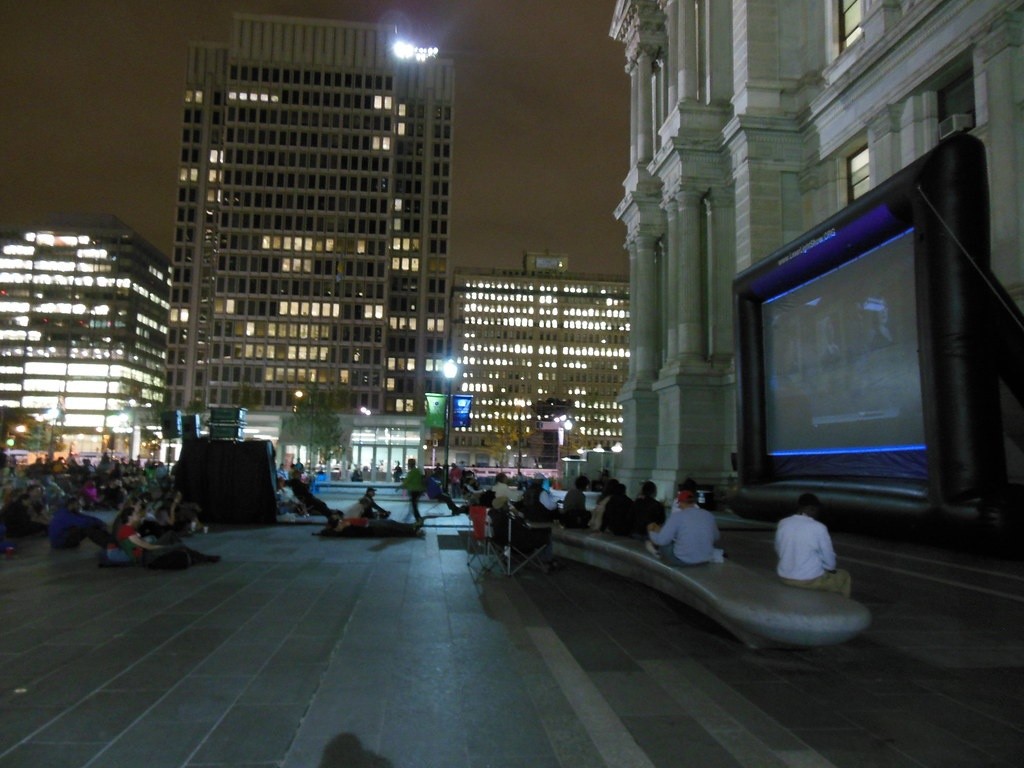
[[414, 517, 423, 532], [203, 552, 221, 562], [452, 507, 464, 516]]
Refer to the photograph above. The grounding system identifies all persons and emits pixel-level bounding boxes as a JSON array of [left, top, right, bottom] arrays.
[[770, 337, 811, 402], [813, 316, 850, 396], [276, 457, 343, 523], [774, 493, 852, 598], [869, 297, 899, 351], [0, 451, 220, 570], [478, 472, 593, 571], [395, 459, 424, 527], [385, 448, 478, 498], [425, 468, 464, 516], [343, 486, 423, 532], [592, 479, 720, 568]]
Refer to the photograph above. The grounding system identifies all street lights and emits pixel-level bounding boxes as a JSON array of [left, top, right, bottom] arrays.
[[440, 358, 458, 492], [554, 413, 571, 459]]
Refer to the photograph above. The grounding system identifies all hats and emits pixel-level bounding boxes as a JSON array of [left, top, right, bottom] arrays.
[[366, 488, 376, 493], [677, 491, 694, 503], [799, 494, 825, 508], [492, 497, 508, 509]]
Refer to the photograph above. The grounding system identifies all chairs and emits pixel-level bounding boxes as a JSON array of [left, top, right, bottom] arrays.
[[423, 492, 445, 516], [484, 509, 553, 579], [466, 504, 506, 578]]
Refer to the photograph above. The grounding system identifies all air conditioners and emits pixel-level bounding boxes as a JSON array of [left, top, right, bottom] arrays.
[[938, 113, 973, 140]]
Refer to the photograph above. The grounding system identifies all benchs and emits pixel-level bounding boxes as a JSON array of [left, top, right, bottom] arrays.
[[551, 528, 872, 651]]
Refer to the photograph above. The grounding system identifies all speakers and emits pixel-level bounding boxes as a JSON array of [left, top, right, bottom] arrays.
[[181, 414, 199, 440], [160, 409, 181, 439]]
